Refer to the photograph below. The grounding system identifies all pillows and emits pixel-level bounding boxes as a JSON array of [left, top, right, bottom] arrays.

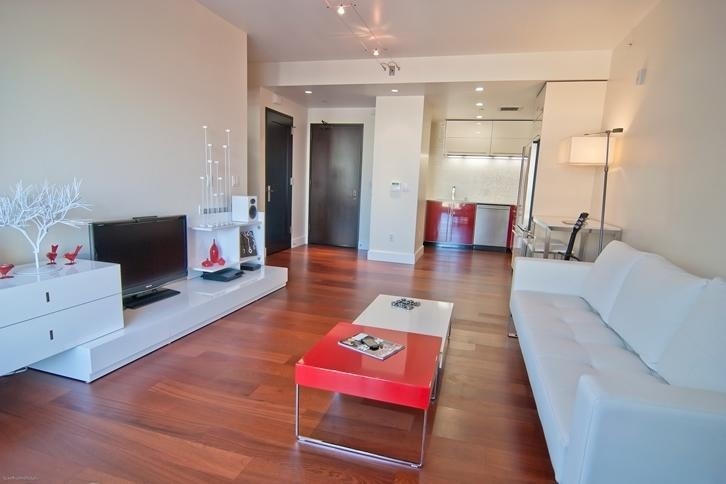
[[577, 240, 726, 391]]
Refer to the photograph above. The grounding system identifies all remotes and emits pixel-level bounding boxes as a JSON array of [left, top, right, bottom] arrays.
[[361, 337, 379, 350]]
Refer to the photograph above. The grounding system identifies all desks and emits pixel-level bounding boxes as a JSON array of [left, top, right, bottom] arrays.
[[24, 263, 288, 384], [530, 214, 622, 259]]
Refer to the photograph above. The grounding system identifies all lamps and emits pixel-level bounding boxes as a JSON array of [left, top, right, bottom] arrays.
[[198, 125, 235, 228], [321, 0, 400, 76], [557, 128, 623, 256]]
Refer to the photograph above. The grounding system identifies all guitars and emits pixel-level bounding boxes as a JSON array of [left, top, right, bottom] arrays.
[[564, 213, 589, 260]]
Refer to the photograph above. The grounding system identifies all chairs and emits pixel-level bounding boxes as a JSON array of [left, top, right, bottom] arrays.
[[516, 224, 568, 260], [512, 225, 566, 257]]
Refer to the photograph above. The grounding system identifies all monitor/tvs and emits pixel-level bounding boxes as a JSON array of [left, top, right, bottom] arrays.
[[88, 215, 187, 309]]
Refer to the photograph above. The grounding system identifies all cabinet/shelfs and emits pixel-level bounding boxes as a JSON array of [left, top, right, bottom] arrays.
[[188, 212, 266, 278], [1, 255, 126, 380], [423, 114, 533, 253]]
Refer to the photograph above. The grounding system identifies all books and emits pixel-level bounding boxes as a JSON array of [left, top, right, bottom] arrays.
[[336, 332, 405, 361]]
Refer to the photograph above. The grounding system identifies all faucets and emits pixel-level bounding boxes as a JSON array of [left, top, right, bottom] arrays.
[[451, 185, 456, 201]]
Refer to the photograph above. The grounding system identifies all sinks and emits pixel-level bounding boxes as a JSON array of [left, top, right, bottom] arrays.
[[442, 201, 465, 210]]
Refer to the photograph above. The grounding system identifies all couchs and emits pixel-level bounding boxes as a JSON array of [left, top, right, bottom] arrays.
[[508, 257, 726, 484]]
